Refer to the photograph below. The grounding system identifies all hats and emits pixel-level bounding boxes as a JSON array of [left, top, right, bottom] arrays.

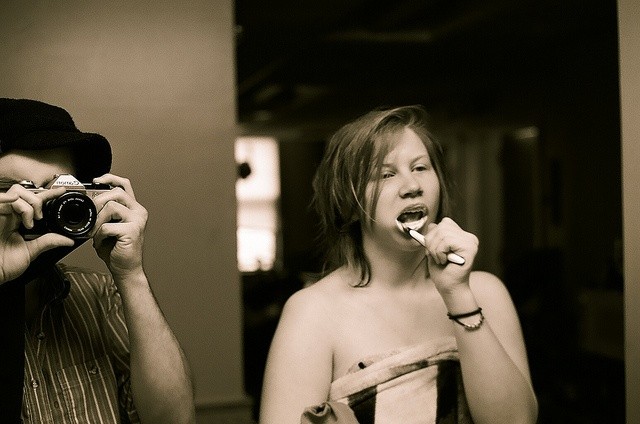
[[0, 98, 112, 190]]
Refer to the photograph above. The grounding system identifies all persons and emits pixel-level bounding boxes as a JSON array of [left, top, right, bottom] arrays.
[[0, 98, 201, 424], [257, 106, 540, 424]]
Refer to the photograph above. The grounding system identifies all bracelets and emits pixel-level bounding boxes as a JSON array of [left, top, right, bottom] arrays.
[[448, 308, 485, 330]]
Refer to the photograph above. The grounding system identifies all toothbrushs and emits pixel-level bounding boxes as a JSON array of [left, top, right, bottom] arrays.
[[402, 222, 466, 265]]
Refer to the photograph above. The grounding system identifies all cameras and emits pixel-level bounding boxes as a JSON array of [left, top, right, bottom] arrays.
[[19, 174, 118, 241]]
